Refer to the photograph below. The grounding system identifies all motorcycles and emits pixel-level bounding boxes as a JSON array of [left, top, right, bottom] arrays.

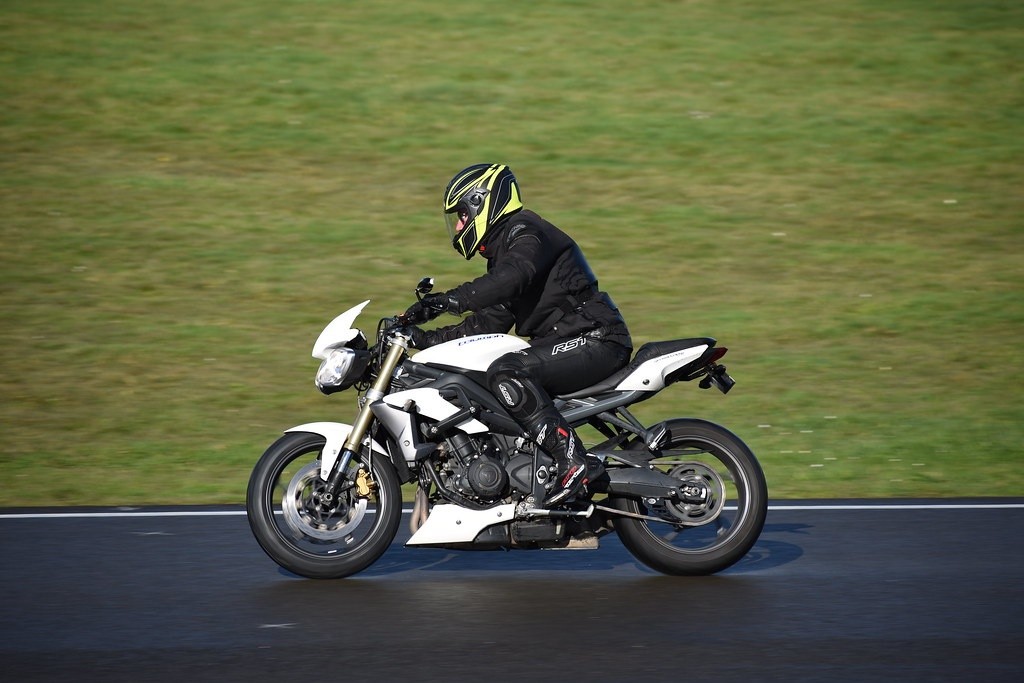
[[246, 275, 770, 582]]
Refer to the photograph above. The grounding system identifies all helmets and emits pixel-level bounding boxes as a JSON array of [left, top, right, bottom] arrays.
[[443, 163, 524, 260]]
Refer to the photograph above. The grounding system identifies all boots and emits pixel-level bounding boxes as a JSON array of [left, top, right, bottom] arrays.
[[522, 406, 606, 508]]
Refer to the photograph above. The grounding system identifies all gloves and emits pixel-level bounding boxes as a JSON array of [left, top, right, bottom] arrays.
[[405, 293, 450, 324], [406, 326, 425, 347]]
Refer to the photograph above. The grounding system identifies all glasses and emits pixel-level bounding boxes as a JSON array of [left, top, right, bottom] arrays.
[[458, 208, 470, 225]]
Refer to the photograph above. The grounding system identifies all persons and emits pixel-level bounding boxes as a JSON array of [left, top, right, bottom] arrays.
[[401, 163, 633, 504]]
